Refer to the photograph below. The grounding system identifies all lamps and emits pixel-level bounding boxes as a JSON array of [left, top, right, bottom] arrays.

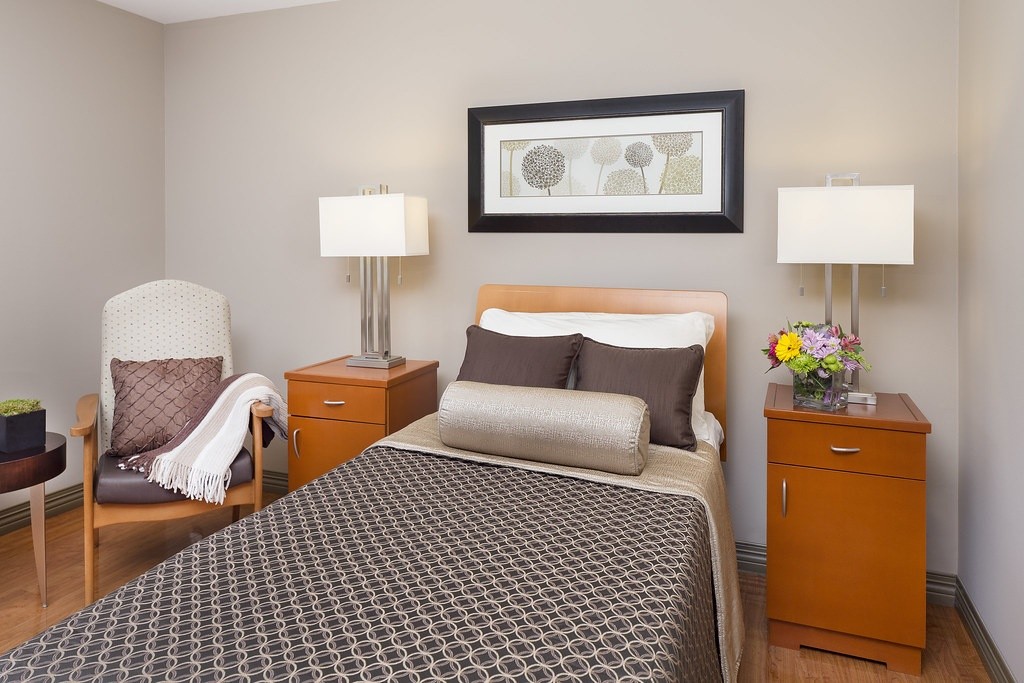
[[318, 183, 430, 369], [777, 173, 915, 406]]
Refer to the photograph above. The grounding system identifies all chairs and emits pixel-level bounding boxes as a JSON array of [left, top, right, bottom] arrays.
[[69, 278, 274, 608]]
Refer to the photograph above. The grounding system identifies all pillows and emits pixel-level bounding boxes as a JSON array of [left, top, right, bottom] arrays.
[[478, 308, 715, 441], [439, 379, 652, 476], [571, 337, 705, 453], [456, 324, 584, 390], [105, 355, 225, 459]]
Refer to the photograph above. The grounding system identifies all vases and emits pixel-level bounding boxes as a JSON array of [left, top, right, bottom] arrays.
[[792, 368, 851, 412]]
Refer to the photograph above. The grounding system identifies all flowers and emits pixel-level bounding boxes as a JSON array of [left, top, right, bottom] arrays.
[[761, 317, 873, 402]]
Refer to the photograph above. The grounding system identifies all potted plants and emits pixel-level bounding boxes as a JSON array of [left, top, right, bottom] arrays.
[[0, 397, 47, 454]]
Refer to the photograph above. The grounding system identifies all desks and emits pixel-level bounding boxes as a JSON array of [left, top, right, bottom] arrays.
[[0, 432, 67, 608]]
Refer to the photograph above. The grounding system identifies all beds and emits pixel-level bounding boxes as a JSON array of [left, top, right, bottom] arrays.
[[0, 282, 745, 682]]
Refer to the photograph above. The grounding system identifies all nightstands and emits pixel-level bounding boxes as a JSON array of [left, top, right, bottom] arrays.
[[764, 382, 933, 677], [283, 354, 439, 494]]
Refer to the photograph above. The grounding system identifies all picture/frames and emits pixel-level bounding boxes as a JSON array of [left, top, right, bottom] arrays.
[[467, 90, 746, 234]]
